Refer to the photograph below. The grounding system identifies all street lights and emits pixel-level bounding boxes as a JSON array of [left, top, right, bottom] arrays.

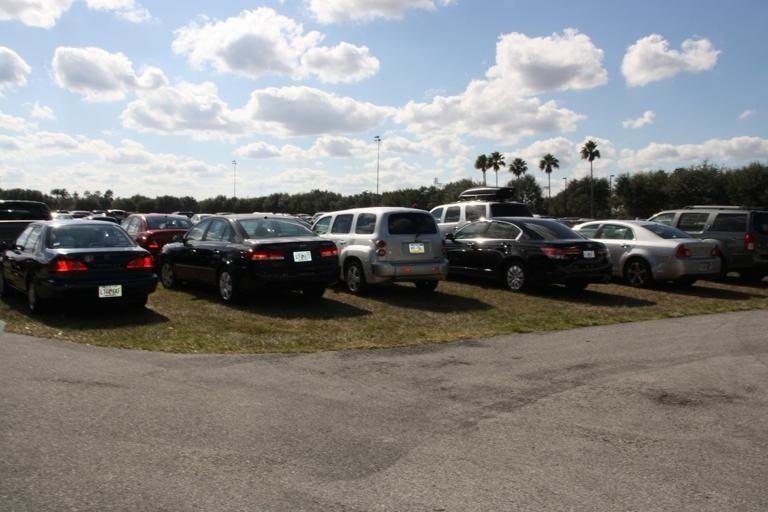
[[232, 160, 236, 197], [374, 136, 380, 194], [562, 177, 567, 196], [610, 175, 615, 190]]
